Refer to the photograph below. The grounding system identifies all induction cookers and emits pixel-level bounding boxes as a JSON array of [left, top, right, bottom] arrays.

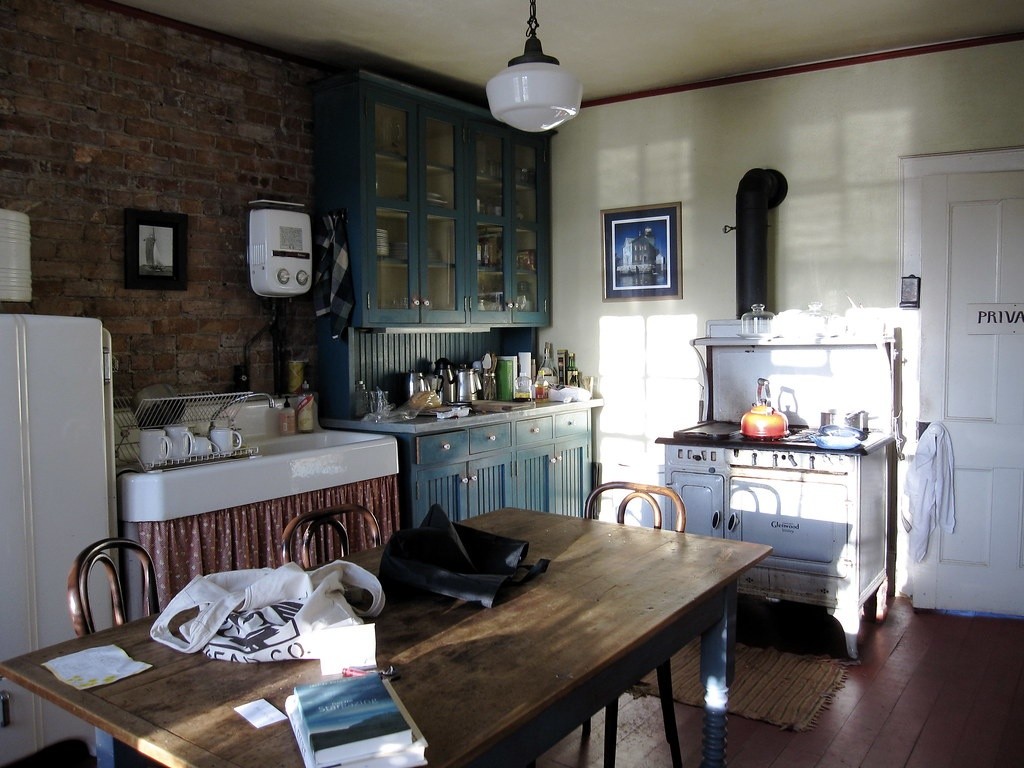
[[674, 420, 809, 447]]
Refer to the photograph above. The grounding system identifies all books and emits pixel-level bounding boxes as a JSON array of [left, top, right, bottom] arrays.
[[283, 671, 428, 768]]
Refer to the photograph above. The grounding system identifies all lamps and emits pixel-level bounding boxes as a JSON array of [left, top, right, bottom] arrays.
[[486, 0, 584, 132]]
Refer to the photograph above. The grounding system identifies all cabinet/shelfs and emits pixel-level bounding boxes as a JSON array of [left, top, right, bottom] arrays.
[[390, 408, 591, 528], [310, 73, 554, 331], [661, 447, 889, 660]]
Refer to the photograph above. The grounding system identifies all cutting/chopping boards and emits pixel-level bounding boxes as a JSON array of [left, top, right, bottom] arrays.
[[472, 399, 536, 412]]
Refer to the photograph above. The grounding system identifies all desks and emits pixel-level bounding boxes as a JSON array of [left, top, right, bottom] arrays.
[[0, 506, 773, 768]]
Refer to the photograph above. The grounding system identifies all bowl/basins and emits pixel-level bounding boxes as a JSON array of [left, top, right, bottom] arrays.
[[129, 383, 187, 427]]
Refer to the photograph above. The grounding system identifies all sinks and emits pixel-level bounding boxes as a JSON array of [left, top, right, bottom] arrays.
[[246, 430, 386, 455]]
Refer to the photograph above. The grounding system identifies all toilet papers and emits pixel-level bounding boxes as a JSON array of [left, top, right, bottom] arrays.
[[518, 351, 531, 379]]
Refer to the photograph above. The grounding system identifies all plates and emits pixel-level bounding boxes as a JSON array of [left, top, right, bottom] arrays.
[[398, 190, 448, 207], [734, 332, 779, 339], [811, 425, 867, 449], [377, 228, 408, 260]]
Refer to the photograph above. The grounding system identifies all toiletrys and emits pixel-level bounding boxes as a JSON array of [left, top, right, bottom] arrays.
[[278, 395, 296, 435]]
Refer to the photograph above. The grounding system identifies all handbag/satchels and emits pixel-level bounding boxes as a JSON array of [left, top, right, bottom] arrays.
[[379, 503, 551, 610], [150, 560, 386, 664]]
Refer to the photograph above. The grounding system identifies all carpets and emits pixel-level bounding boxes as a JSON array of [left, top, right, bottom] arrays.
[[627, 637, 849, 731]]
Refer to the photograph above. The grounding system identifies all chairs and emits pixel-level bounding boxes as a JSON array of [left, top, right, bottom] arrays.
[[67, 538, 159, 637], [281, 503, 381, 567], [578, 480, 688, 768]]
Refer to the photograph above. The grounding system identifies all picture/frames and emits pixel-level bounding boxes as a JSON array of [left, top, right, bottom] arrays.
[[124, 208, 188, 291], [603, 201, 683, 301]]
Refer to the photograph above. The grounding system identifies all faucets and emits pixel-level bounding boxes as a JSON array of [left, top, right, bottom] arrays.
[[208, 392, 274, 440]]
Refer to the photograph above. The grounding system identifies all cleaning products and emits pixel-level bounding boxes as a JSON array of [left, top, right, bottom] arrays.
[[296, 380, 315, 434]]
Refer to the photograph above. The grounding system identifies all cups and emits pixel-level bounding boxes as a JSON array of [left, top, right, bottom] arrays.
[[211, 428, 242, 455], [289, 360, 304, 394], [194, 436, 220, 456], [164, 426, 195, 462], [139, 429, 173, 466]]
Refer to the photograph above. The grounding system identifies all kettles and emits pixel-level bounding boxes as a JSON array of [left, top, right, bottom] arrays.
[[426, 374, 444, 405], [409, 371, 431, 398], [739, 378, 791, 440], [454, 363, 482, 402], [433, 358, 458, 403]]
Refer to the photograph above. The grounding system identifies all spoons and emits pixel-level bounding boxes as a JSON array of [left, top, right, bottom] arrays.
[[483, 354, 492, 372]]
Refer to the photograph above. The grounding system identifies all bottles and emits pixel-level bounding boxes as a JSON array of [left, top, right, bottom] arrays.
[[534, 371, 549, 403], [482, 373, 496, 401], [570, 371, 579, 387], [375, 174, 389, 207], [370, 391, 389, 413], [478, 281, 534, 312], [354, 381, 369, 417], [537, 342, 559, 389], [476, 157, 503, 272], [739, 302, 833, 333], [512, 373, 532, 403], [297, 384, 315, 434], [566, 352, 578, 387]]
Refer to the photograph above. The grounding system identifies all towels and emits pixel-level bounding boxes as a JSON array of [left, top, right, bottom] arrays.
[[902, 420, 957, 564]]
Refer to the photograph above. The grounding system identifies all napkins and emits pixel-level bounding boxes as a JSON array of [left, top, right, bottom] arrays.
[[40, 643, 155, 690]]
